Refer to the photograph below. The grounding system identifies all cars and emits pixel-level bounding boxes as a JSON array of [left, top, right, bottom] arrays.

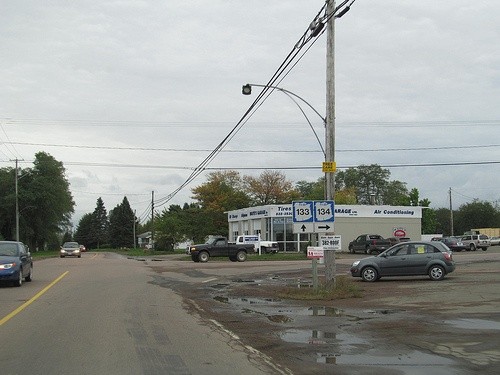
[[0, 240, 34, 288], [349, 239, 457, 283], [432, 235, 467, 252], [60, 242, 82, 258], [488, 236, 500, 246], [79, 245, 87, 253]]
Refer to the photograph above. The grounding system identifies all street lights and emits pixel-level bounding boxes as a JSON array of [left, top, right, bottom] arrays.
[[242, 82, 335, 201]]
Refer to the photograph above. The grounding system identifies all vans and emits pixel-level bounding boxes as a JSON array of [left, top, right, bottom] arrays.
[[460, 234, 491, 251]]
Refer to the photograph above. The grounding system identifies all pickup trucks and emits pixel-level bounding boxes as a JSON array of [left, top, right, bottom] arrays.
[[348, 234, 392, 255], [226, 234, 280, 255], [189, 236, 255, 264]]
